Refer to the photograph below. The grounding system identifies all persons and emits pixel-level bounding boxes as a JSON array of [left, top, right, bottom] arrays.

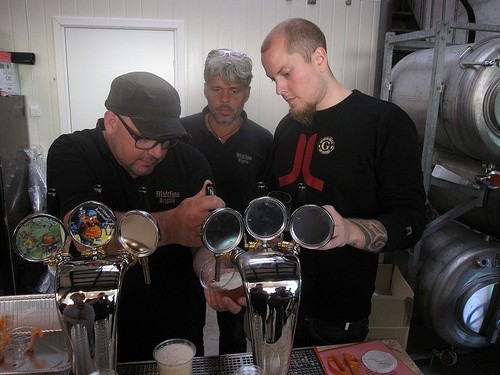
[[47, 72, 247, 360], [249, 283, 299, 345], [56, 291, 117, 346], [172, 50, 281, 357], [260, 18, 426, 350]]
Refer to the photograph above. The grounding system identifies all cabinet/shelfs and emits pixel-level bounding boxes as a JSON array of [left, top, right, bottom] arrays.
[[380, 20, 499, 361]]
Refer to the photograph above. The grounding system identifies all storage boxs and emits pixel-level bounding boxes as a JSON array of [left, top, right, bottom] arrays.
[[370, 265, 414, 348]]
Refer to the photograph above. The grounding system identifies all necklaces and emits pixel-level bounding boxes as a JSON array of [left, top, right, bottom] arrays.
[[208, 114, 242, 139]]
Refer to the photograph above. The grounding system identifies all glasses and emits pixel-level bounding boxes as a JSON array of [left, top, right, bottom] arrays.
[[115, 114, 182, 150], [208, 49, 248, 60]]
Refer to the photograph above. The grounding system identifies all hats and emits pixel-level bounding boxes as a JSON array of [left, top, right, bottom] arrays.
[[105, 72, 186, 139]]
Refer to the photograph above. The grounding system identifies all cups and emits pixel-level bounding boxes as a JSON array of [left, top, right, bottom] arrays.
[[153, 338, 197, 375], [0, 318, 37, 363], [198, 251, 246, 305], [234, 364, 264, 375]]
